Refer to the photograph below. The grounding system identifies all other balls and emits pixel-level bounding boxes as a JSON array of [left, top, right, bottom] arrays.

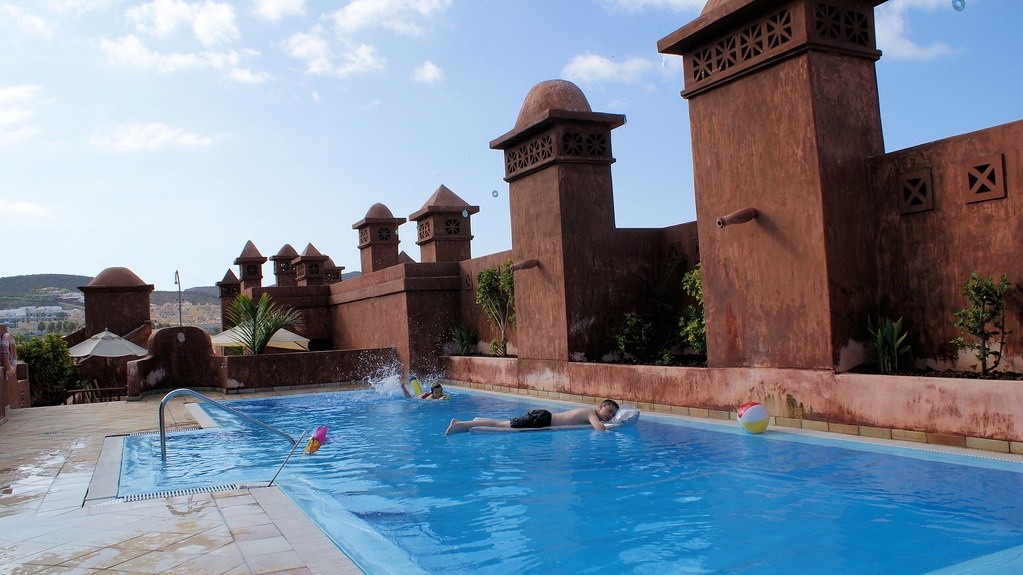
[[736, 401, 770, 434]]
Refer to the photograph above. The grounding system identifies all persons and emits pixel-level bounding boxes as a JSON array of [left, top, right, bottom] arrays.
[[445, 400, 618, 437], [398, 376, 446, 400]]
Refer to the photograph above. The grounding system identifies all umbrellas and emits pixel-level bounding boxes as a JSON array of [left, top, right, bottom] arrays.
[[211, 318, 310, 352], [68, 327, 148, 401]]
